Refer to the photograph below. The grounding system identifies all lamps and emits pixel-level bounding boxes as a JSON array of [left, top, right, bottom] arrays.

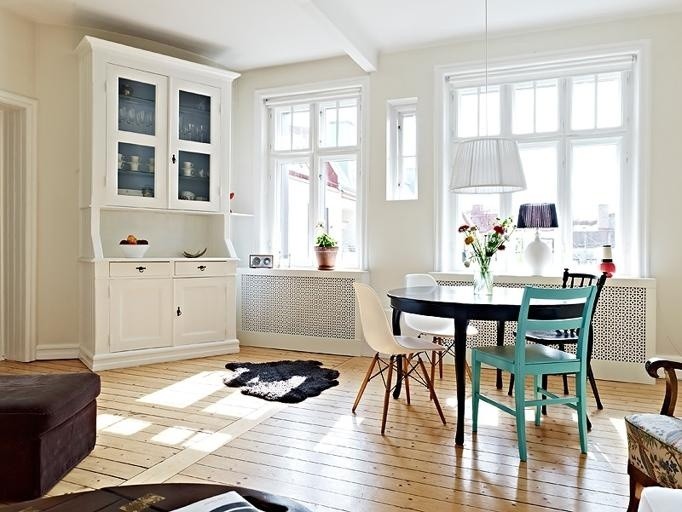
[[448, 136, 524, 195], [517, 203, 558, 278]]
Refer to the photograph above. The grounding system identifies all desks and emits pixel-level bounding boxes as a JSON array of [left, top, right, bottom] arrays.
[[387, 285, 598, 447], [1, 485, 308, 512]]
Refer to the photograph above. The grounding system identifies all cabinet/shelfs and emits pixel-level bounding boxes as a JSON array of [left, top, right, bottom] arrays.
[[74, 35, 240, 213], [77, 209, 240, 372]]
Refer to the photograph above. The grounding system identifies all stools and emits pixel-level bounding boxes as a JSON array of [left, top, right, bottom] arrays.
[[0, 371, 102, 501], [455, 213, 518, 290]]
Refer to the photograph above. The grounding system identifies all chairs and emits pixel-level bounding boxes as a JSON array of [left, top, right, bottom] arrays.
[[508, 269, 608, 411], [471, 285, 598, 462], [400, 273, 476, 401], [623, 358, 682, 512], [350, 282, 445, 435]]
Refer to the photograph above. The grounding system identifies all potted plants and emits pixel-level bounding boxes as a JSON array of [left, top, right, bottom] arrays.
[[314, 226, 338, 270]]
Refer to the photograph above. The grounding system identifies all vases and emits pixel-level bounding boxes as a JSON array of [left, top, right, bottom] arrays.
[[472, 265, 493, 296]]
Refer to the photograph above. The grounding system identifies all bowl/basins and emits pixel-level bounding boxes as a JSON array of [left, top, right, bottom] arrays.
[[121, 244, 149, 258]]
